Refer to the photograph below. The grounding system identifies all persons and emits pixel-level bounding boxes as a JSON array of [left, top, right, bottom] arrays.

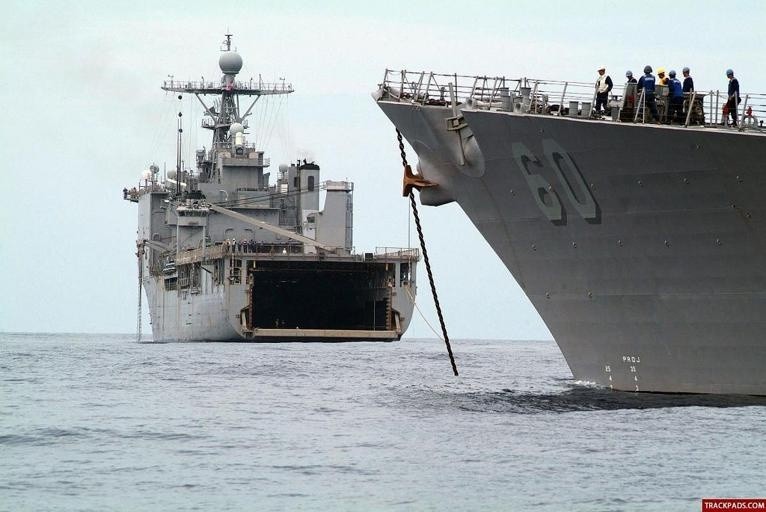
[[717, 69, 741, 127], [283, 248, 287, 255], [595, 68, 613, 111], [626, 66, 694, 125], [227, 238, 247, 253]]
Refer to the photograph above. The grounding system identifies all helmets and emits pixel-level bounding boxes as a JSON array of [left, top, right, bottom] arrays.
[[667, 69, 676, 76], [682, 66, 690, 73], [725, 68, 734, 75], [625, 70, 632, 78], [656, 67, 665, 75], [595, 64, 606, 72], [642, 64, 653, 74]]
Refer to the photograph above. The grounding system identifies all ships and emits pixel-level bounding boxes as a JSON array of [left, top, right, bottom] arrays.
[[120, 32, 423, 344], [369, 65, 766, 407]]
